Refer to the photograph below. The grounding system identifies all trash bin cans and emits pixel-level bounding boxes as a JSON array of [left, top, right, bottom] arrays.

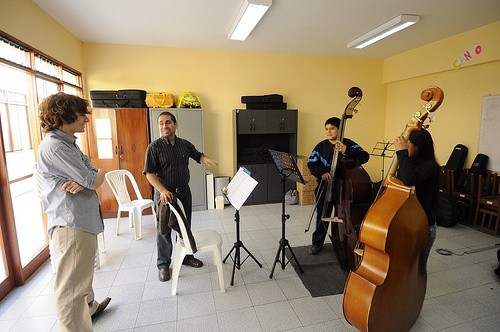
[[215, 176, 231, 206]]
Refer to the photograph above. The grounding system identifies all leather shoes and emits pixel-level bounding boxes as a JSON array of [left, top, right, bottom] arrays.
[[159, 268, 170, 281], [182, 256, 203, 268]]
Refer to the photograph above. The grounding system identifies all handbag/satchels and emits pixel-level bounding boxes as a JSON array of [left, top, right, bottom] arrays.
[[284, 189, 299, 205], [146, 92, 174, 108], [179, 91, 201, 108]]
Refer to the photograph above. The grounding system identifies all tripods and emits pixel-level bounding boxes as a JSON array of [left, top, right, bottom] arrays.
[[267, 150, 308, 279], [222, 167, 263, 287]]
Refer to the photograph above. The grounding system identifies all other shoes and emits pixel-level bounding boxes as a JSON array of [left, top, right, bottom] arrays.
[[308, 244, 321, 254], [90, 297, 111, 320]]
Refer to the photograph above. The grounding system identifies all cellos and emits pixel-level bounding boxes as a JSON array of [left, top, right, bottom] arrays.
[[320, 87, 376, 273], [342, 85, 445, 332]]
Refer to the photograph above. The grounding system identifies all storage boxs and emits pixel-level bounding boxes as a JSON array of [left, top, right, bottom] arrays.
[[297, 154, 318, 205]]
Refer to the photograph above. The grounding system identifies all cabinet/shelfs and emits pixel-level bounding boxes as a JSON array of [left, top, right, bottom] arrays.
[[87, 109, 152, 218], [148, 108, 207, 212], [232, 109, 299, 206]]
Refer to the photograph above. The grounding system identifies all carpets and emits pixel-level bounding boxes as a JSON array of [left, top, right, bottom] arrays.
[[282, 242, 352, 299]]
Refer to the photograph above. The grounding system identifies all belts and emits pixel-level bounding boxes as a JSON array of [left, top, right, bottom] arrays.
[[166, 186, 189, 193]]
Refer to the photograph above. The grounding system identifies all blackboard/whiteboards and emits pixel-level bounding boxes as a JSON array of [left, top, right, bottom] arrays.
[[478, 95, 500, 172]]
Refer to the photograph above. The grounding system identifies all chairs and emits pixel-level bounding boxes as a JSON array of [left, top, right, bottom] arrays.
[[440, 168, 500, 235], [168, 198, 227, 295], [105, 170, 157, 240]]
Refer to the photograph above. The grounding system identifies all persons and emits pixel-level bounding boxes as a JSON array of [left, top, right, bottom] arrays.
[[306, 117, 370, 255], [142, 112, 218, 282], [36, 92, 111, 332], [393, 128, 441, 280]]
